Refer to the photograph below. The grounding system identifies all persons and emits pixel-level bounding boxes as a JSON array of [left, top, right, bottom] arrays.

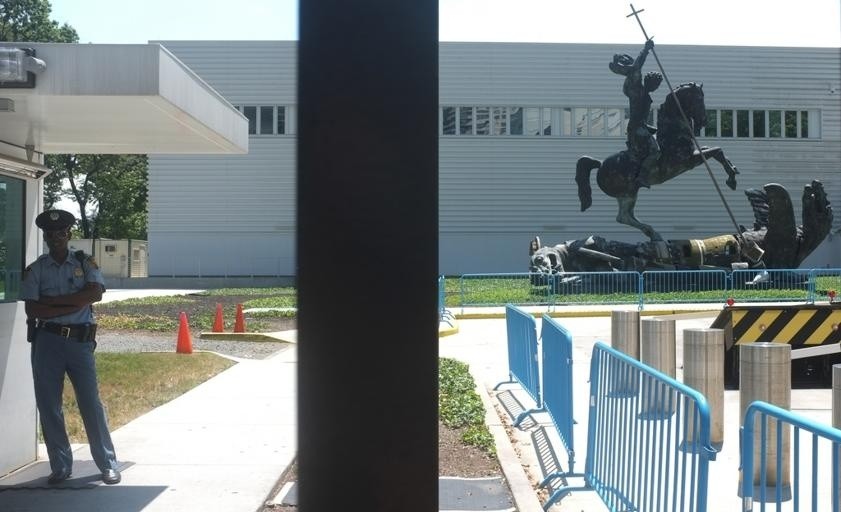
[[17, 211, 121, 484], [610, 35, 663, 194]]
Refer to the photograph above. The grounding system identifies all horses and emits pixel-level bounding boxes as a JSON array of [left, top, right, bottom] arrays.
[[575, 81, 740, 243]]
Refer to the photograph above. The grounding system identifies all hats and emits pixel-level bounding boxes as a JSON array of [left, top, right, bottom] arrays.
[[35, 209, 76, 230]]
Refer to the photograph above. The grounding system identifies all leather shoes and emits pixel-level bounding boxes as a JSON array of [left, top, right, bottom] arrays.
[[102, 467, 121, 484], [49, 466, 72, 484]]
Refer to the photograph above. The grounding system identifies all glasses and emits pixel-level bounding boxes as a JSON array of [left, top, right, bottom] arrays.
[[43, 228, 69, 240]]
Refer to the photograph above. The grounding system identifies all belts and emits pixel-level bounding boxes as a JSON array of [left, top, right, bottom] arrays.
[[38, 320, 90, 339]]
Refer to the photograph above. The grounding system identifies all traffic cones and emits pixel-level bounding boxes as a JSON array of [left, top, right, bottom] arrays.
[[172, 311, 195, 355], [210, 300, 228, 333], [231, 301, 246, 333]]
